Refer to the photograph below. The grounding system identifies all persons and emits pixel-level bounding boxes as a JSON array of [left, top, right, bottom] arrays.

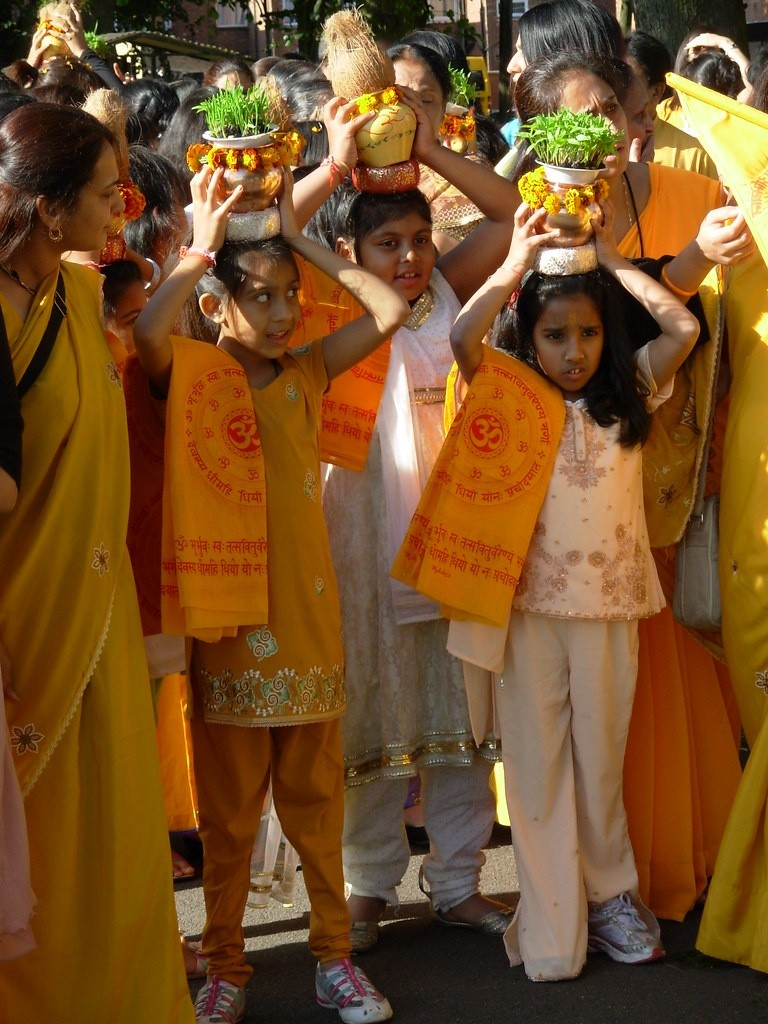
[[134, 164, 411, 1024], [0, 105, 196, 1024], [0, 0, 768, 1024], [451, 202, 701, 980]]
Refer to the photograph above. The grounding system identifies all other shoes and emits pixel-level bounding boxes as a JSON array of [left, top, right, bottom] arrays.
[[185, 939, 208, 978], [418, 864, 516, 936], [349, 901, 386, 951]]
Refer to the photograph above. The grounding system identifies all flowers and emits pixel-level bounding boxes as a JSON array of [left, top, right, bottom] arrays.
[[348, 90, 398, 121], [437, 116, 475, 139], [110, 184, 146, 227], [184, 129, 306, 172], [517, 167, 611, 213]]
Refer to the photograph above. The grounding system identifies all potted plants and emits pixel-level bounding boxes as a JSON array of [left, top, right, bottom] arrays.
[[443, 64, 477, 117], [191, 76, 281, 148], [514, 107, 623, 193]]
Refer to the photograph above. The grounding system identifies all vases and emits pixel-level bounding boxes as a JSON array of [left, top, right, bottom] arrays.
[[440, 134, 467, 155], [101, 235, 124, 264], [204, 164, 286, 214], [348, 101, 419, 170], [530, 199, 604, 251]]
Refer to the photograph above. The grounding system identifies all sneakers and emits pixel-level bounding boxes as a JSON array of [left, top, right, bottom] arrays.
[[586, 892, 665, 963], [314, 961, 392, 1024], [193, 975, 245, 1024]]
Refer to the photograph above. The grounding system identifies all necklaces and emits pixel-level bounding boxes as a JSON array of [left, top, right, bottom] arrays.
[[623, 172, 644, 258], [272, 362, 279, 377], [622, 175, 633, 227], [0, 264, 37, 295]]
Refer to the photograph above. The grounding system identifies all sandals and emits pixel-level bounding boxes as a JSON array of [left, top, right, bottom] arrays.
[[172, 852, 195, 883]]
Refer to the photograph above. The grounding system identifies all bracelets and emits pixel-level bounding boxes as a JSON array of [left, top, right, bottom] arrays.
[[321, 156, 350, 190], [144, 258, 161, 295], [497, 266, 522, 310], [726, 44, 738, 55], [180, 246, 216, 268], [662, 264, 700, 296]]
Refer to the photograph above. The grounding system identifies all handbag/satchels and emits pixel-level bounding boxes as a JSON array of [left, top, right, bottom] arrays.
[[671, 494, 722, 631]]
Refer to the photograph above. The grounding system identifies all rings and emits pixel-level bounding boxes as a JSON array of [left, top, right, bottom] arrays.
[[65, 27, 71, 32]]
